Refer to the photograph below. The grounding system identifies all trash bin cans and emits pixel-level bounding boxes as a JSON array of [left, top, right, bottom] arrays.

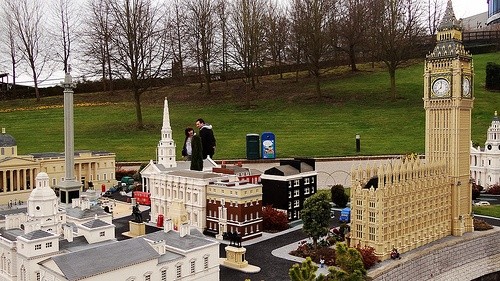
[[246, 134, 259, 161], [261, 133, 276, 159]]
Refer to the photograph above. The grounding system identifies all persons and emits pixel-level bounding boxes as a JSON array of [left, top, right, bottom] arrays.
[[196, 119, 216, 159], [182, 128, 197, 161]]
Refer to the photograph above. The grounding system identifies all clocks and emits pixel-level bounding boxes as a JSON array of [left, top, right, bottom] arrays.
[[431, 76, 451, 98], [462, 78, 470, 96]]
